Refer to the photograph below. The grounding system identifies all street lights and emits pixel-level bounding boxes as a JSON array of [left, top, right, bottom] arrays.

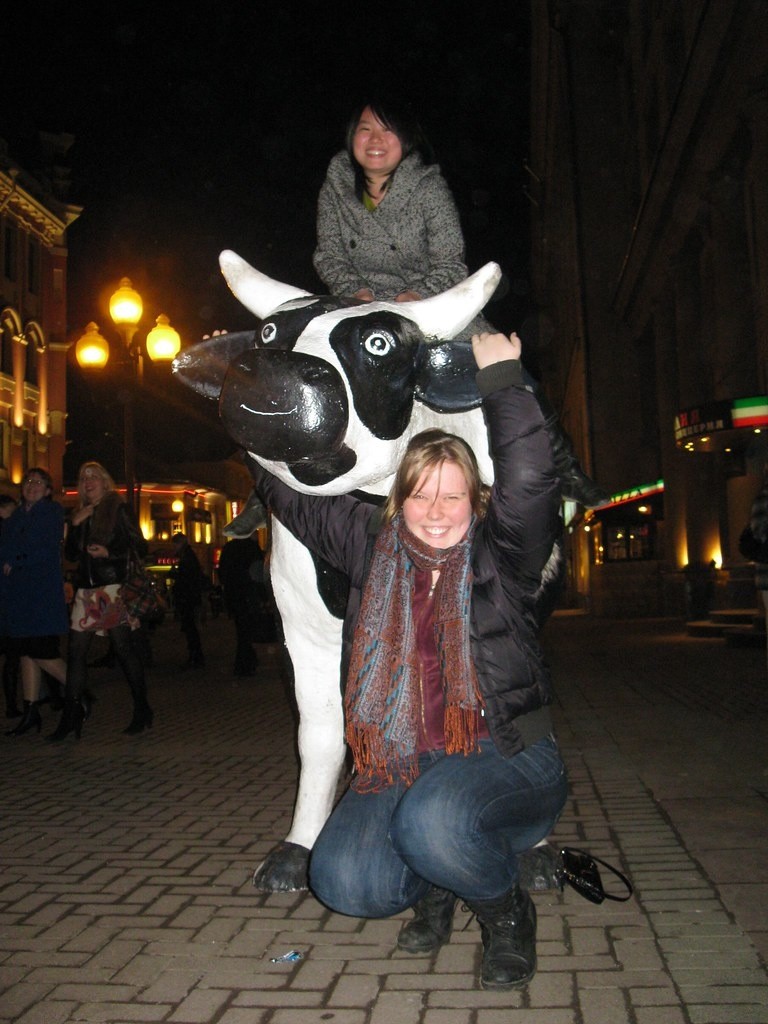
[[75, 277, 181, 512]]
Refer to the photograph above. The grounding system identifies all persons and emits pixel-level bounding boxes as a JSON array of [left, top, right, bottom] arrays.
[[739, 485, 768, 612], [203, 329, 569, 992], [221, 531, 277, 679], [221, 89, 612, 540], [1, 462, 204, 743]]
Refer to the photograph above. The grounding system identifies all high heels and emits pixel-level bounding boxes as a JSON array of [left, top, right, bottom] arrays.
[[45, 704, 84, 741], [121, 707, 153, 736]]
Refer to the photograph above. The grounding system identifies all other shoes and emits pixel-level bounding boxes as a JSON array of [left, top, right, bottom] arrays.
[[234, 662, 256, 674], [5, 708, 24, 719], [50, 696, 65, 712]]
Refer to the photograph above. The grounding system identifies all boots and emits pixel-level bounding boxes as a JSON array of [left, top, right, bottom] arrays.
[[222, 486, 266, 539], [534, 385, 610, 508], [3, 699, 41, 737], [82, 692, 95, 722], [461, 881, 537, 991], [179, 641, 205, 670], [398, 885, 456, 951]]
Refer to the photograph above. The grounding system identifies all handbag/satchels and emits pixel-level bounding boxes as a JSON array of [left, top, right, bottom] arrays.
[[120, 547, 168, 620], [558, 846, 633, 904]]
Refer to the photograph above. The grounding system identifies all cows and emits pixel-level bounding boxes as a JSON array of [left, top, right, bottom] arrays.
[[168, 248, 565, 897]]
